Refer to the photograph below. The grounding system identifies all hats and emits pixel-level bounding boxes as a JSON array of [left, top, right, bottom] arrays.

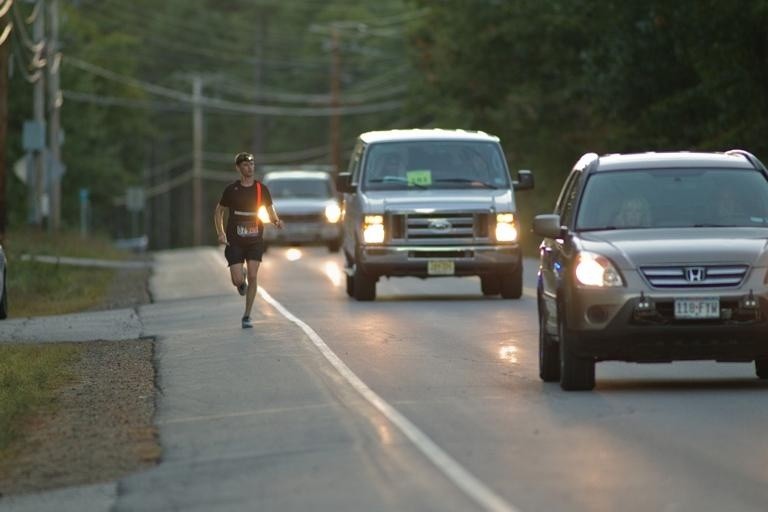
[[236, 152, 253, 164]]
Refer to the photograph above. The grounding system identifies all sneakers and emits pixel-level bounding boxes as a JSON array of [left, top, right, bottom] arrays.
[[238, 267, 248, 296], [242, 314, 252, 328]]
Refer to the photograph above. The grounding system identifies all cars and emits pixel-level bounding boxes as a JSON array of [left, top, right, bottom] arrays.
[[259, 169, 344, 251], [0, 243, 10, 320]]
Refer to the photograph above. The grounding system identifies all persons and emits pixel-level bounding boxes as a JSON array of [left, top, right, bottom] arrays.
[[704, 184, 746, 225], [211, 152, 285, 329], [461, 153, 495, 183], [380, 152, 406, 178], [616, 194, 653, 230]]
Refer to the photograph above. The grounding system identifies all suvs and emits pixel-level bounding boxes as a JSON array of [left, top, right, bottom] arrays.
[[332, 126, 536, 303], [532, 146, 768, 390]]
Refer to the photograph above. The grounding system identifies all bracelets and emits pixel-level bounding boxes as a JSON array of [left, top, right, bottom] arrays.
[[273, 220, 279, 225]]
